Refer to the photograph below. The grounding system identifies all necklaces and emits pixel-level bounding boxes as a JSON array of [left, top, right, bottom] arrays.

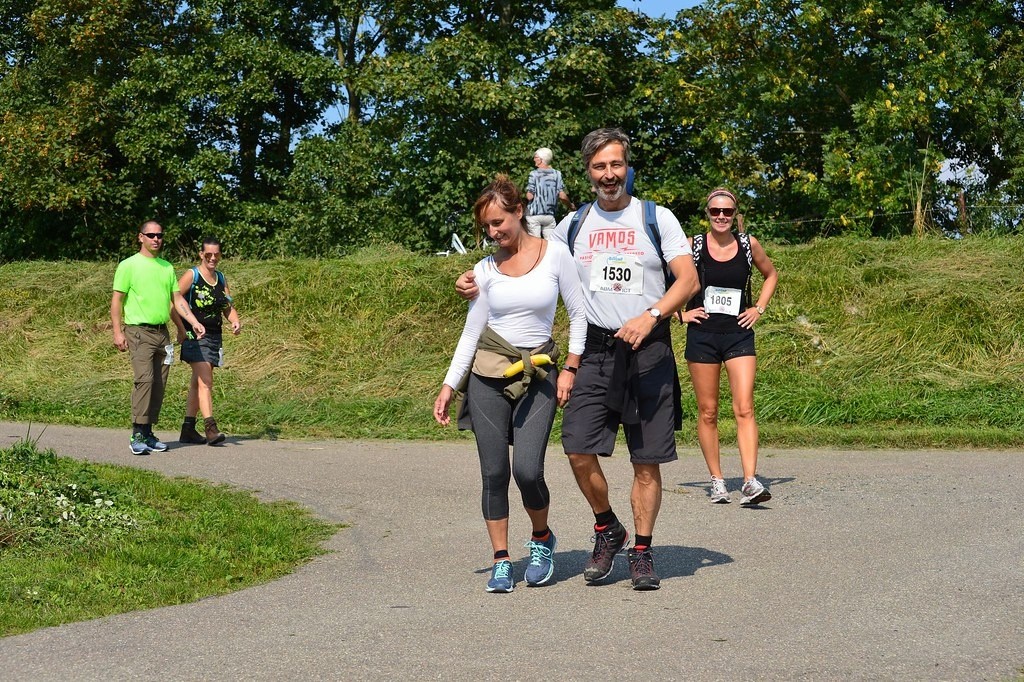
[[491, 239, 543, 277]]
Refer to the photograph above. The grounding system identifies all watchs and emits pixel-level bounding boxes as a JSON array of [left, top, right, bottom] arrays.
[[647, 308, 661, 324], [563, 365, 577, 372], [754, 305, 764, 314]]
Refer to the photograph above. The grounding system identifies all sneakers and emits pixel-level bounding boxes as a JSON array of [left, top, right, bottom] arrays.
[[484, 559, 515, 593], [129, 433, 149, 455], [740, 478, 772, 506], [583, 520, 630, 580], [524, 531, 556, 584], [710, 474, 731, 504], [627, 546, 660, 590], [143, 432, 168, 452]]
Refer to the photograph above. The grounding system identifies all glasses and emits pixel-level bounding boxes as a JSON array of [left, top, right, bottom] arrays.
[[708, 207, 736, 217], [142, 233, 165, 239]]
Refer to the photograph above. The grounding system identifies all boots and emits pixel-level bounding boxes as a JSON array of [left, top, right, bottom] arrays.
[[204, 420, 226, 445], [179, 419, 208, 444]]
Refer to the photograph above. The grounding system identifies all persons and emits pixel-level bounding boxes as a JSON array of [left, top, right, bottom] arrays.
[[527, 147, 577, 240], [111, 221, 205, 454], [454, 130, 701, 590], [433, 174, 588, 591], [171, 239, 241, 444], [675, 188, 777, 505]]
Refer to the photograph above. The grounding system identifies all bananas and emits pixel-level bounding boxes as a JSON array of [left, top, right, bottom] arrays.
[[503, 354, 555, 378]]
